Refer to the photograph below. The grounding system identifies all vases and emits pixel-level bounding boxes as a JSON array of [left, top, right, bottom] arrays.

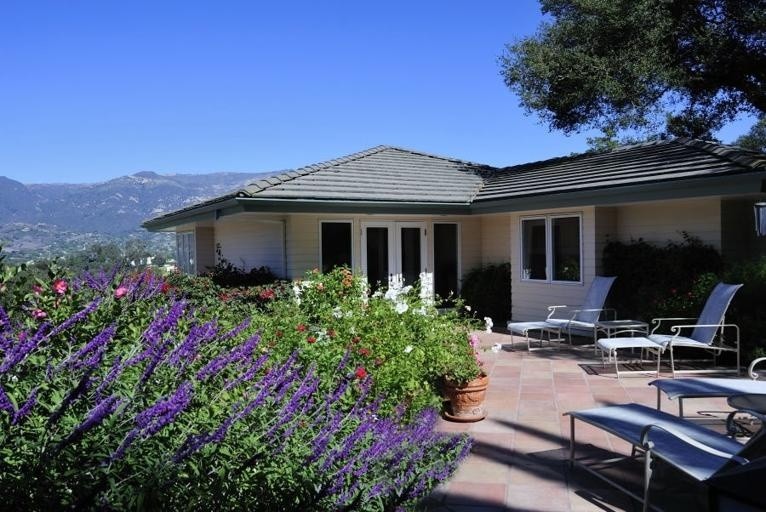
[[441, 368, 490, 423], [427, 372, 450, 400]]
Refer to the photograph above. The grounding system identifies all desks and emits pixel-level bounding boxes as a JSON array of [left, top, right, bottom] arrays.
[[725, 390, 766, 439], [586, 315, 650, 365]]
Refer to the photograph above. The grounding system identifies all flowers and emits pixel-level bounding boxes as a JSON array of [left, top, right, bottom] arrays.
[[461, 311, 503, 377]]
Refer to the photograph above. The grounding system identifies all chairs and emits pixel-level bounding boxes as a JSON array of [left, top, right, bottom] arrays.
[[645, 354, 766, 428], [595, 281, 748, 383], [505, 269, 622, 355], [561, 402, 766, 512]]
